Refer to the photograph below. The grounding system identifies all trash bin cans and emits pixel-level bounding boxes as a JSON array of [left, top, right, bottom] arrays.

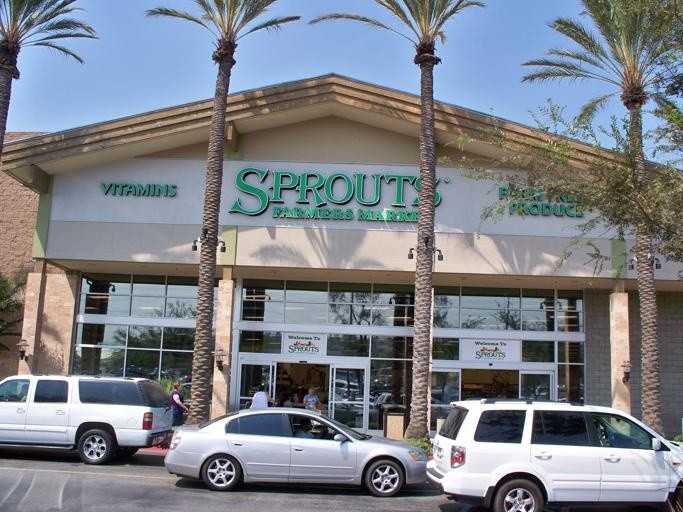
[[382, 404, 406, 440]]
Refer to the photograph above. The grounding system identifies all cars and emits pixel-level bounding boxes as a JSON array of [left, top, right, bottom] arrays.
[[425, 396, 683, 512], [335, 366, 445, 412], [164, 407, 428, 497]]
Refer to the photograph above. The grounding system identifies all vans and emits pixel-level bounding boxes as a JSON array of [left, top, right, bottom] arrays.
[[0, 374, 174, 464]]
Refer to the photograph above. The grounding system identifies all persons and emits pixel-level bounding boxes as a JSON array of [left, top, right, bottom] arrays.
[[294, 417, 329, 438], [250, 385, 274, 408], [171, 381, 188, 426], [20, 385, 29, 401], [304, 388, 321, 409]]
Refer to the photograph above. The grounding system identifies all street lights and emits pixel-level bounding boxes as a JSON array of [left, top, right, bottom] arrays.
[[87, 278, 115, 373]]
